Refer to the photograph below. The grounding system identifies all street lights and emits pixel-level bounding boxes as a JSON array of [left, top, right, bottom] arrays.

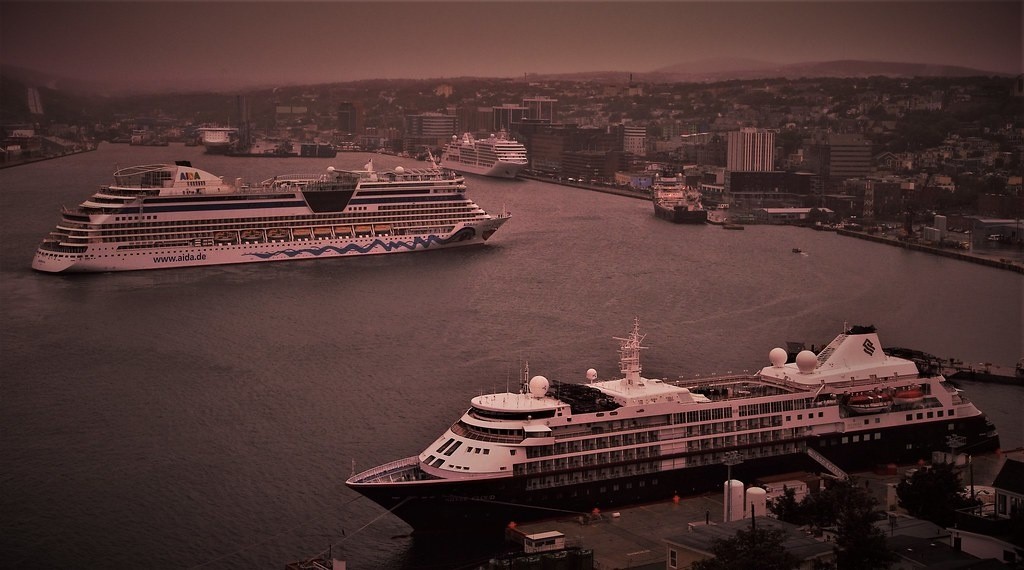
[[721, 450, 744, 522]]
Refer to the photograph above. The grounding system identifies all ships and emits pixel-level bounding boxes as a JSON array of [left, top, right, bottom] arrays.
[[29, 159, 513, 276], [438, 126, 529, 179], [344, 315, 1002, 541]]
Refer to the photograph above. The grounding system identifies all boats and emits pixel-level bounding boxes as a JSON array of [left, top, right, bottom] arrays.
[[334, 226, 351, 236], [374, 224, 390, 234], [313, 227, 331, 236], [214, 233, 233, 242], [847, 394, 893, 414], [293, 229, 310, 238], [355, 225, 371, 235], [267, 230, 287, 239], [242, 230, 262, 240], [892, 389, 924, 406], [651, 173, 707, 224]]
